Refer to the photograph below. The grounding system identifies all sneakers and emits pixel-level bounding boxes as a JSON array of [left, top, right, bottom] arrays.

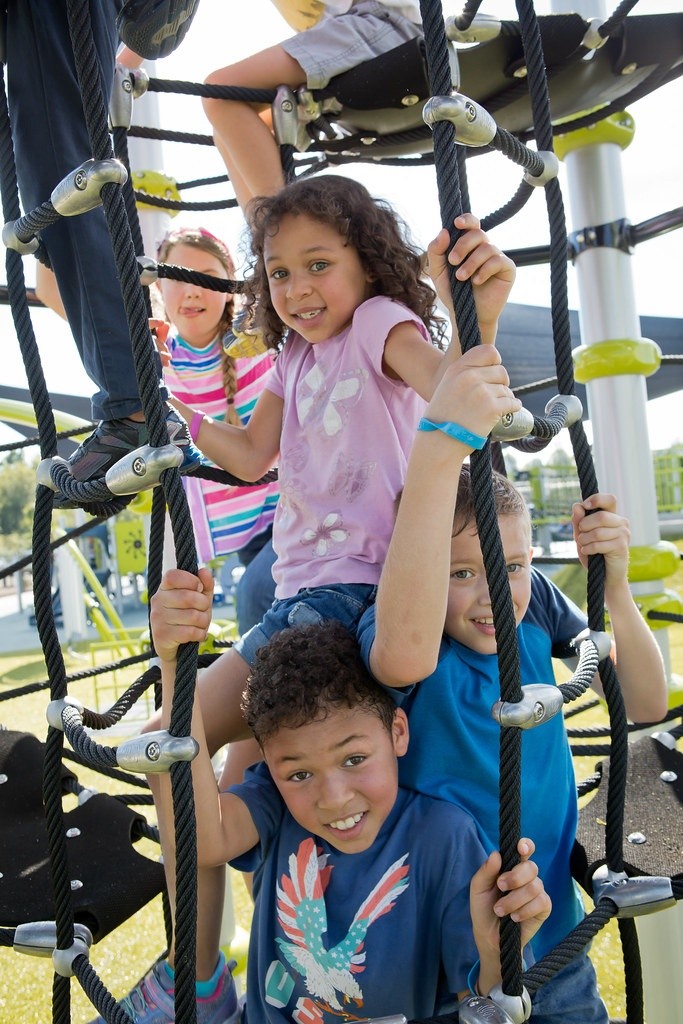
[[66, 404, 204, 485], [87, 953, 241, 1024], [222, 291, 274, 357]]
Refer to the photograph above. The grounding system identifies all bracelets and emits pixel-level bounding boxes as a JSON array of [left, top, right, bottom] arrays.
[[416, 416, 489, 453], [466, 956, 529, 1009], [188, 407, 206, 447]]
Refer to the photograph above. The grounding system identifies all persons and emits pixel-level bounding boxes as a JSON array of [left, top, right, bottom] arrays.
[[147, 567, 554, 1023], [0, 0, 173, 489], [355, 341, 671, 1024], [145, 224, 283, 570], [84, 170, 519, 1024], [113, 0, 430, 248]]
